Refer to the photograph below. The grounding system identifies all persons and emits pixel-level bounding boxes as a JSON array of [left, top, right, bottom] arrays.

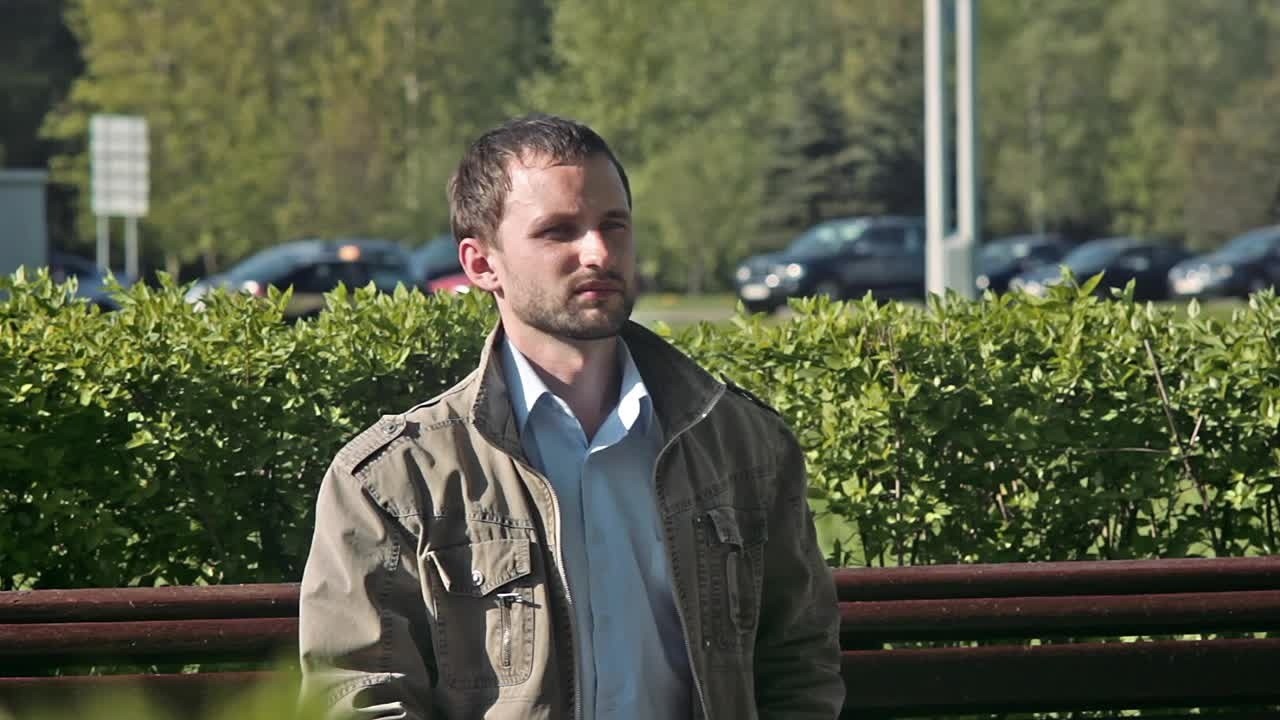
[[299, 116, 847, 719]]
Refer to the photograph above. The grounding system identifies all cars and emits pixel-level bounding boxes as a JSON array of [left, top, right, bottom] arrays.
[[1009, 233, 1198, 306], [732, 210, 926, 314], [184, 233, 421, 324], [977, 230, 1075, 299], [1166, 223, 1280, 307]]
